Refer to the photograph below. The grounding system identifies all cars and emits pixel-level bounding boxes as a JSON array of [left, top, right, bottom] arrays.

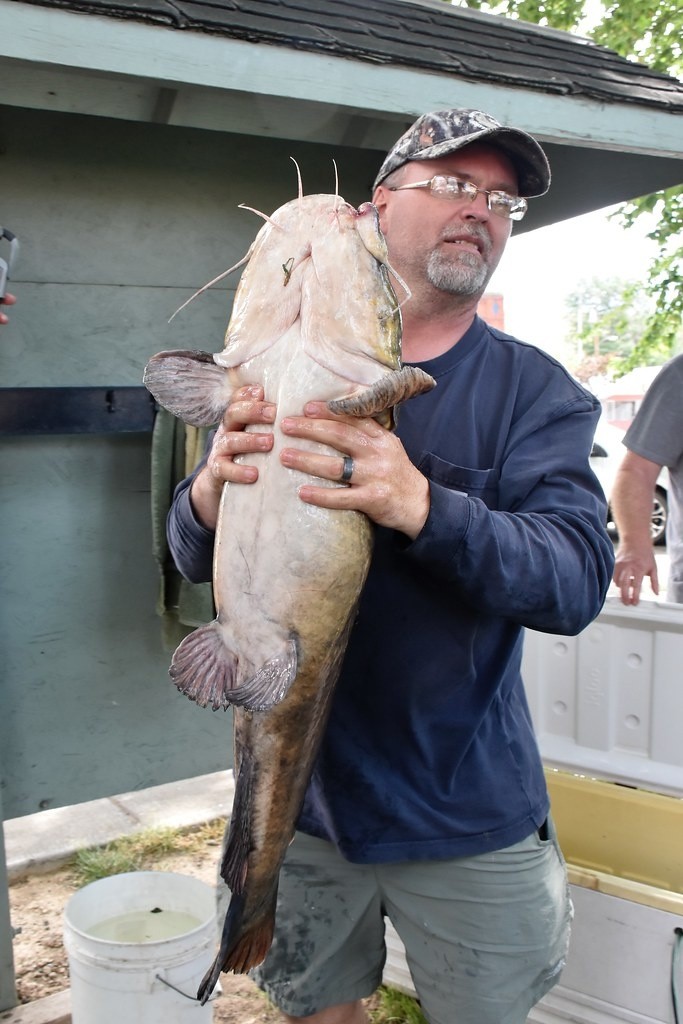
[[588, 423, 672, 545]]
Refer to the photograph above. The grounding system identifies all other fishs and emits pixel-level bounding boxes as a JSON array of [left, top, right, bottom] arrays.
[[143, 156, 439, 1007]]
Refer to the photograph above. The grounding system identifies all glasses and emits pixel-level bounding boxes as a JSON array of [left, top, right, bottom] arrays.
[[389, 174, 528, 221]]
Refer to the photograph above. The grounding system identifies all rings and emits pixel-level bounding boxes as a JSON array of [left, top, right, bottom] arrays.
[[341, 456, 354, 479]]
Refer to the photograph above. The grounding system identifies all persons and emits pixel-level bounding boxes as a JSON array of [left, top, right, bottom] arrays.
[[165, 106, 615, 1024], [611, 354, 683, 605]]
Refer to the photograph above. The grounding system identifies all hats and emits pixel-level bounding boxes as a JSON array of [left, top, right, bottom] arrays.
[[373, 108, 552, 199]]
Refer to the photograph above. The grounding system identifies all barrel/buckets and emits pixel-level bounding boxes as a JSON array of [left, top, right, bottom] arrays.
[[62, 871, 220, 1024]]
